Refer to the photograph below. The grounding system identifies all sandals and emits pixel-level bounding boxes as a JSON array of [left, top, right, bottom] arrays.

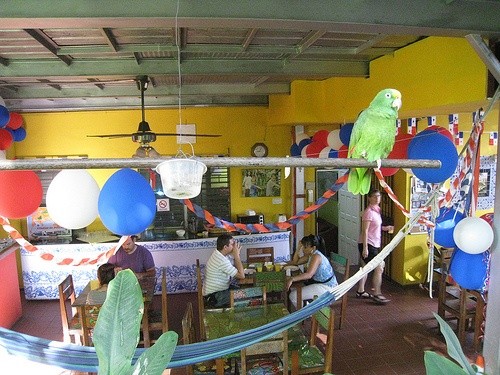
[[356, 290, 373, 299], [372, 294, 390, 303]]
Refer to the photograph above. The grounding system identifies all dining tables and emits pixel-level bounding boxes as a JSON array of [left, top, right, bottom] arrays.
[[436, 266, 489, 352], [71, 276, 157, 348], [201, 303, 307, 375], [229, 263, 306, 311]]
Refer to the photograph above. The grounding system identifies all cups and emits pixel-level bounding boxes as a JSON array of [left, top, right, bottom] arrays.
[[275, 263, 281, 272], [257, 263, 263, 272], [249, 211, 255, 216], [286, 268, 291, 277]]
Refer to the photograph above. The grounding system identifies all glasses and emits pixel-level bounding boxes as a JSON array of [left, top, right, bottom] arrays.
[[370, 195, 381, 197]]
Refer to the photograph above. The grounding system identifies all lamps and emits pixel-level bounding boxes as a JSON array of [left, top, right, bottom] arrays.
[[132, 144, 160, 158]]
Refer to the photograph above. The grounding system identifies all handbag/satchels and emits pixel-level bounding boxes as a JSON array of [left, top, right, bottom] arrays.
[[205, 292, 218, 307], [304, 274, 334, 286]]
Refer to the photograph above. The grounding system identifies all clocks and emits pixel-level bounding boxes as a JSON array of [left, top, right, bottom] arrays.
[[251, 143, 269, 158]]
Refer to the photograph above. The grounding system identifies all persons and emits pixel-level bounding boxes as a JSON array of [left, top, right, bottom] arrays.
[[286, 235, 339, 311], [202, 234, 245, 308], [107, 235, 156, 279], [355, 189, 391, 304], [242, 169, 281, 196]]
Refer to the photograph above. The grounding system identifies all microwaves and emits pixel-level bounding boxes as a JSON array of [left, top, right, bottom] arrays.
[[236, 213, 264, 225]]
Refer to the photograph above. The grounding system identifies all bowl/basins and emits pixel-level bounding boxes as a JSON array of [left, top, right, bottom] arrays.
[[175, 229, 185, 237]]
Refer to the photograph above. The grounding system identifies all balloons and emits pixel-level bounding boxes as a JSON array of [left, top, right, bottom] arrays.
[[98, 167, 157, 236], [290, 124, 459, 183], [427, 206, 496, 290], [0, 169, 43, 219], [0, 96, 26, 150], [46, 169, 100, 230]]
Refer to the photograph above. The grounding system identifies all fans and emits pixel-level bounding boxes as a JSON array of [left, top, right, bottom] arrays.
[[86, 75, 222, 143]]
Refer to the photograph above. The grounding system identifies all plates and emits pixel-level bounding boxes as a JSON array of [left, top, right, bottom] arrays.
[[284, 266, 300, 271]]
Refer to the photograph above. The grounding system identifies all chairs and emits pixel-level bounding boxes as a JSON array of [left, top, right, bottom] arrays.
[[433, 248, 479, 348], [58, 268, 168, 346], [182, 246, 349, 375]]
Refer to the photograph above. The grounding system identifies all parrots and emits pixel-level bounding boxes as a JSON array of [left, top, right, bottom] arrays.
[[345, 86, 403, 196]]
